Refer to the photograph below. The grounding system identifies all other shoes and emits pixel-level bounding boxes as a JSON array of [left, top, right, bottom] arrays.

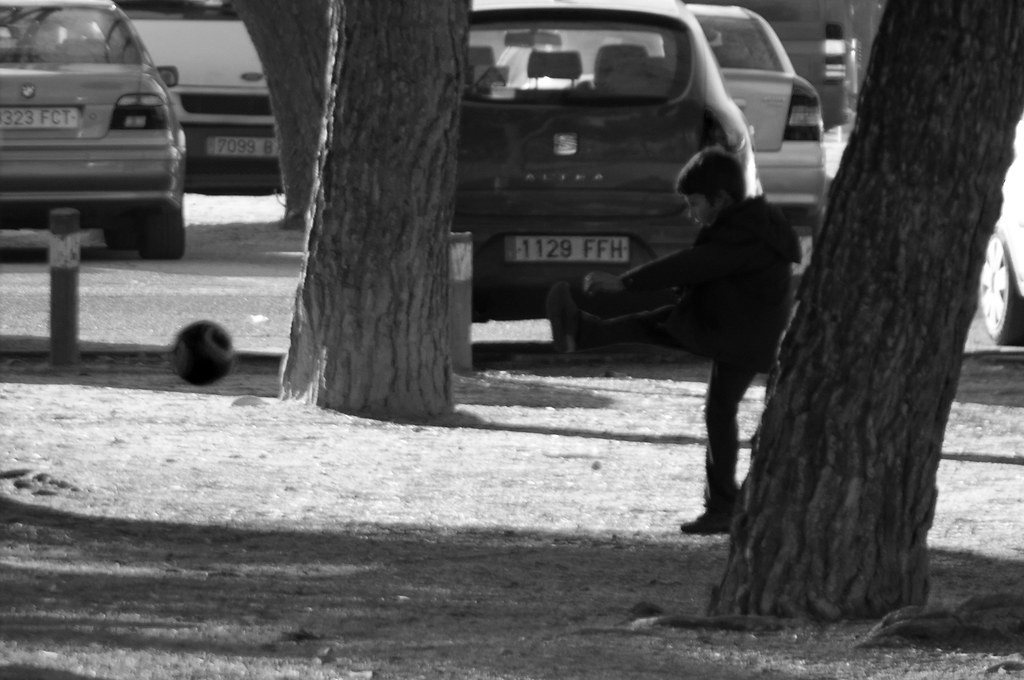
[[680, 502, 730, 534], [546, 280, 582, 354]]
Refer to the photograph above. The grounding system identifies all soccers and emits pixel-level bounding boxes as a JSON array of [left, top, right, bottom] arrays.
[[175, 319, 235, 387]]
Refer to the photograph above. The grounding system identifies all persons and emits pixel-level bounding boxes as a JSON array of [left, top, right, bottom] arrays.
[[671, 146, 802, 535]]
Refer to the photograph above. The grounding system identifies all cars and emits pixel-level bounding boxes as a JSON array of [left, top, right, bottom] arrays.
[[681, 5, 829, 228], [0, 2, 186, 261], [454, 0, 763, 321], [966, 113, 1024, 351]]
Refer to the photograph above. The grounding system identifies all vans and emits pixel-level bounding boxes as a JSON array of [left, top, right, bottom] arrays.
[[113, 1, 284, 198]]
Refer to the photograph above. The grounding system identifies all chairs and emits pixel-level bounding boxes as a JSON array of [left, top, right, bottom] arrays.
[[593, 41, 650, 87]]
